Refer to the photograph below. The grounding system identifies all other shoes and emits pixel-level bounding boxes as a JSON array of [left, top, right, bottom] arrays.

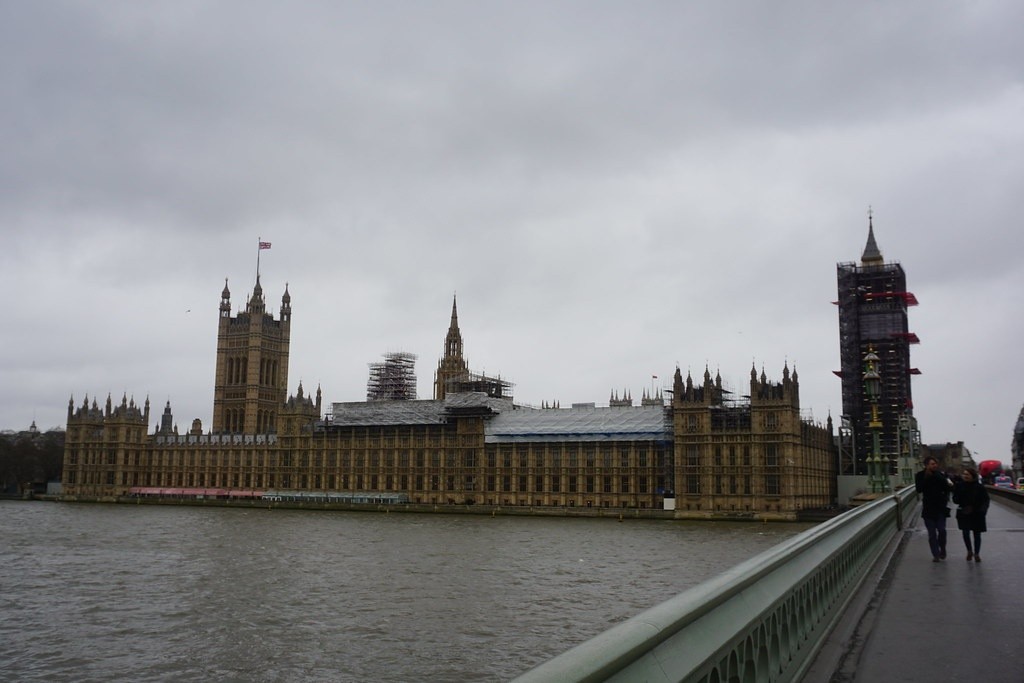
[[967, 551, 973, 561], [974, 553, 980, 563], [933, 551, 940, 562], [940, 547, 947, 559]]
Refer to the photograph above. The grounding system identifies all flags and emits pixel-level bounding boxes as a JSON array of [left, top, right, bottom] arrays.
[[260, 242, 271, 249]]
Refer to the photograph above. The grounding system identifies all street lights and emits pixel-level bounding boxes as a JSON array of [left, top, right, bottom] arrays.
[[861, 344, 891, 493]]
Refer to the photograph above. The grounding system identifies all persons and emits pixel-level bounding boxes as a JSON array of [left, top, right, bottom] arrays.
[[952, 467, 989, 564], [914, 458, 951, 563]]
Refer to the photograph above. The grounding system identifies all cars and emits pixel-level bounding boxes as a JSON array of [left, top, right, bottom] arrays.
[[1016, 478, 1024, 490], [981, 471, 1006, 484], [995, 476, 1013, 488]]
